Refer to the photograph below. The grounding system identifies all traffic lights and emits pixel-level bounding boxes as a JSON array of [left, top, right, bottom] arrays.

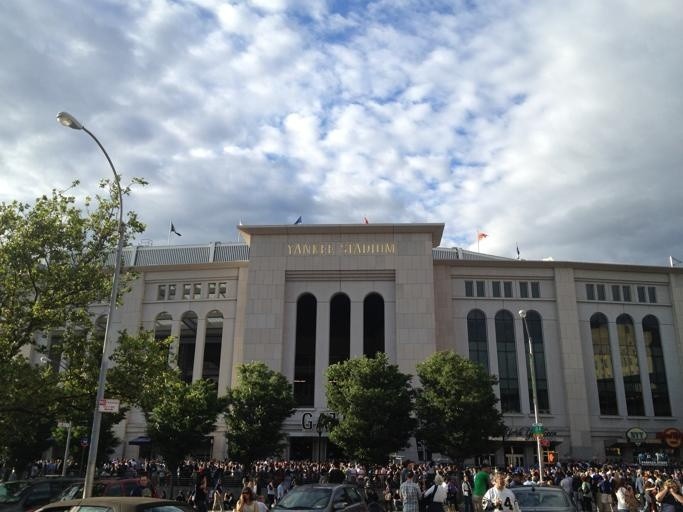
[[547, 451, 557, 463]]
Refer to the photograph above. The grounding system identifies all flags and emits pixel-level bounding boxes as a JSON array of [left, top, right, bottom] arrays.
[[516, 244, 520, 256], [364, 217, 368, 225], [169, 222, 182, 236], [477, 230, 488, 241], [293, 216, 301, 225]]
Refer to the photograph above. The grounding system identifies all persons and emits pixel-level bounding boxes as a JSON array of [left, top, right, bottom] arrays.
[[0, 453, 682, 511]]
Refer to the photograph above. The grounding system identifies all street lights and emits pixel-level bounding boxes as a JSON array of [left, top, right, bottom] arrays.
[[314, 423, 323, 472], [54, 110, 125, 497], [38, 355, 74, 476], [517, 310, 543, 484]]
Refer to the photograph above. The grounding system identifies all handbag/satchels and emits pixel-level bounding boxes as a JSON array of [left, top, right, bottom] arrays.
[[385, 494, 391, 500], [421, 494, 433, 504]]
